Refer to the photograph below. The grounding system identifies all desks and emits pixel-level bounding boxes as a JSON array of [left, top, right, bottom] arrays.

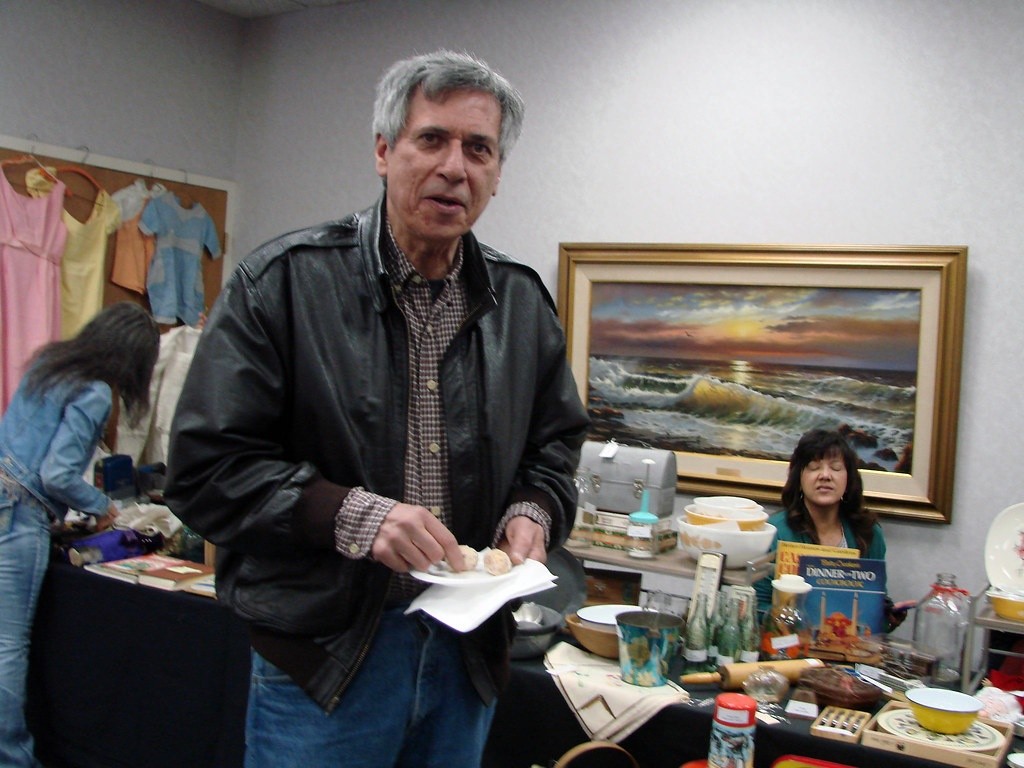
[[26, 539, 970, 768]]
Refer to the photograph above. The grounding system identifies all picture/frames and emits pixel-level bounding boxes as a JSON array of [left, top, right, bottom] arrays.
[[557, 241, 969, 524]]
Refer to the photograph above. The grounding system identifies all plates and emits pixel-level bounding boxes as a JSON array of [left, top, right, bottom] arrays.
[[984, 503, 1024, 597], [877, 710, 1006, 751], [405, 553, 511, 589]]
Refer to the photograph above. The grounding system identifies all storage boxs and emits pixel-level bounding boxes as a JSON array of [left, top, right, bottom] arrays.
[[567, 506, 678, 554]]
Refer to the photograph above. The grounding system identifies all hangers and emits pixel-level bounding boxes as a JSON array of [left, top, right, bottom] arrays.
[[0, 132, 198, 208], [188, 306, 208, 330]]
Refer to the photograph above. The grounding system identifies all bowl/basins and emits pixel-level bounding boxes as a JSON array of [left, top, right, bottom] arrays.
[[675, 495, 777, 569], [986, 591, 1024, 622], [565, 604, 658, 658], [505, 602, 563, 661], [904, 688, 985, 735]]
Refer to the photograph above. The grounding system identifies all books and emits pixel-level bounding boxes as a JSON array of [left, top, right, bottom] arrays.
[[772, 540, 885, 665], [84, 552, 214, 590]]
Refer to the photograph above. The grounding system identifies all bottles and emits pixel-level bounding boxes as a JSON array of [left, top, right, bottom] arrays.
[[626, 489, 658, 559], [707, 694, 757, 768], [743, 664, 789, 703], [760, 573, 812, 662], [913, 572, 972, 688], [679, 578, 760, 676], [564, 466, 596, 548]]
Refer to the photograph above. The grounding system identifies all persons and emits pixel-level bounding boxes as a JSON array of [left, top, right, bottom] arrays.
[[0, 303, 161, 768], [163, 50, 594, 768], [751, 429, 917, 634]]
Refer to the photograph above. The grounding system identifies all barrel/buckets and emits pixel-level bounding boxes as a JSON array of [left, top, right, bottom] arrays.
[[615, 611, 686, 688]]
